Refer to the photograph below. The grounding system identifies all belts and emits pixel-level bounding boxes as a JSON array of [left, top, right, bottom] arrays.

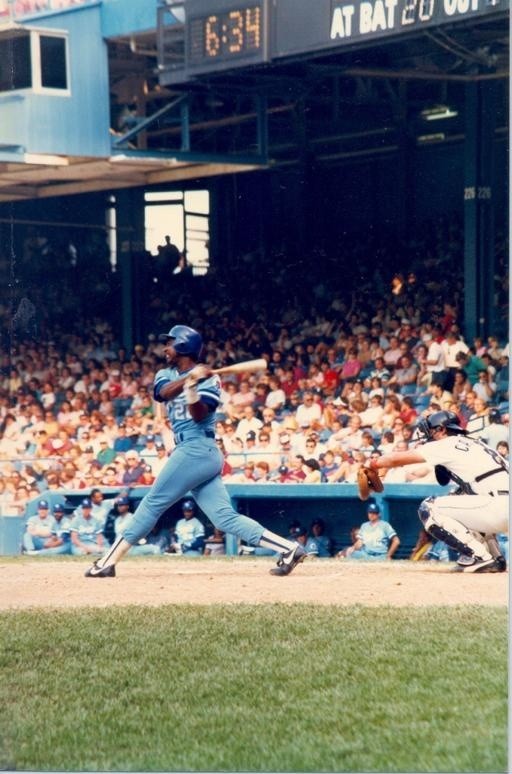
[[173, 430, 215, 445], [489, 490, 508, 496]]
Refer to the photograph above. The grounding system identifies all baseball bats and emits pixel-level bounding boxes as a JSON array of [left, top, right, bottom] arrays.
[[192, 359, 269, 378]]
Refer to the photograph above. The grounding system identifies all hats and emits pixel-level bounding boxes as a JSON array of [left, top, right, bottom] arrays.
[[183, 501, 196, 511], [289, 520, 299, 529], [118, 500, 130, 506], [367, 503, 379, 513], [311, 518, 324, 526], [38, 499, 91, 512]]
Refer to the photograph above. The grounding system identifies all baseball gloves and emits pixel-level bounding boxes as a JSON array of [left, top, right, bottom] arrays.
[[357, 466, 383, 501]]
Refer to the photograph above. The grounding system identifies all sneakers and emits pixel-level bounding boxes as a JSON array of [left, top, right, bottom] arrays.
[[270, 546, 307, 575], [450, 554, 507, 573], [85, 558, 115, 577]]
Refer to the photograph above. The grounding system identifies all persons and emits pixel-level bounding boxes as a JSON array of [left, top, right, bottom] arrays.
[[0, 208, 512, 557], [362, 409, 511, 576], [83, 322, 310, 579]]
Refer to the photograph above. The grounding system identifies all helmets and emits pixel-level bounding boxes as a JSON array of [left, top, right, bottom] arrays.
[[159, 325, 202, 361], [419, 410, 467, 440]]
